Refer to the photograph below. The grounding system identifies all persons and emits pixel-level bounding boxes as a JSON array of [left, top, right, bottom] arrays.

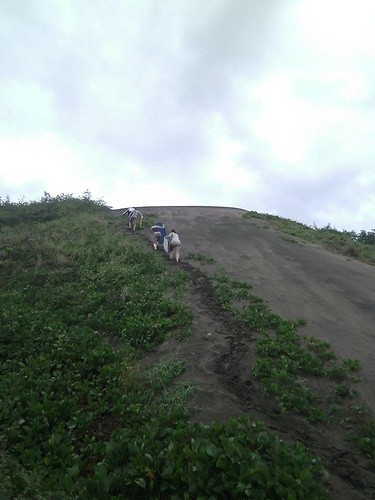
[[152, 223, 167, 249], [128, 209, 143, 230], [164, 230, 182, 262], [123, 207, 136, 228]]
